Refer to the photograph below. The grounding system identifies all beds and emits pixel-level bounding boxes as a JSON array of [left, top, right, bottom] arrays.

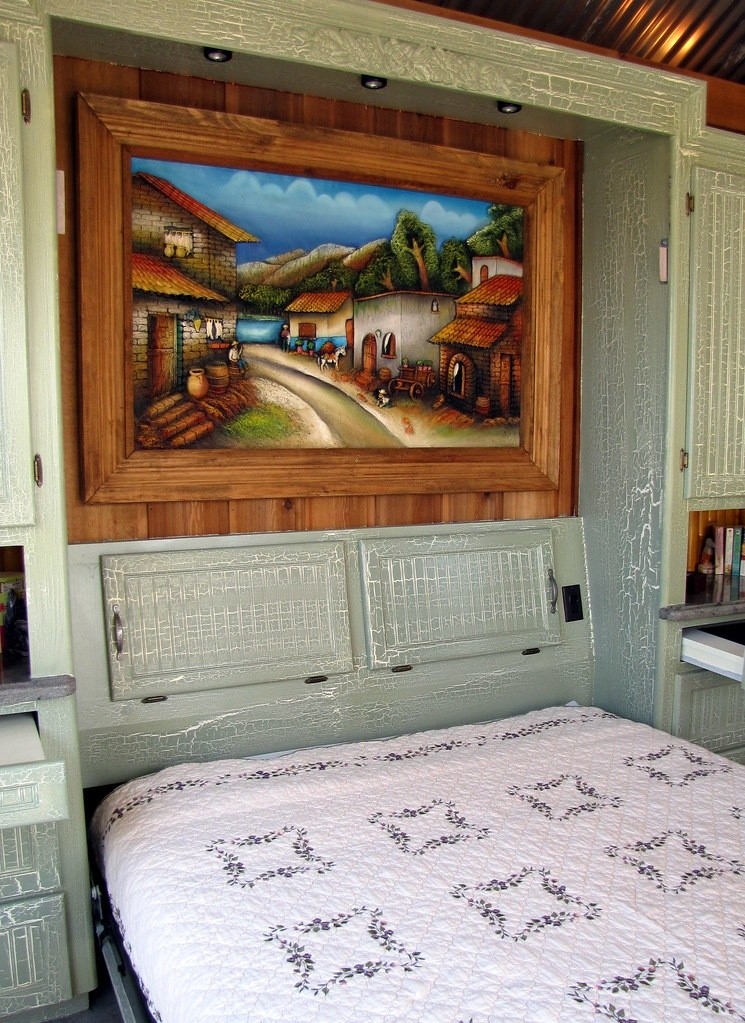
[[68, 514, 744, 1022]]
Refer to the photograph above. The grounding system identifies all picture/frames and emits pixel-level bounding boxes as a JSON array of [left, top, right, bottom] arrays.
[[76, 90, 568, 504]]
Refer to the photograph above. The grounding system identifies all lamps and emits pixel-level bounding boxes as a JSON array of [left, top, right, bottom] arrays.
[[494, 99, 523, 115], [204, 47, 233, 64], [359, 73, 388, 91]]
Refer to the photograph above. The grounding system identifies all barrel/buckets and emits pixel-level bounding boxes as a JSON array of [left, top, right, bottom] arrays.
[[379, 369, 391, 383], [475, 396, 491, 416], [206, 361, 229, 395], [229, 362, 245, 380]]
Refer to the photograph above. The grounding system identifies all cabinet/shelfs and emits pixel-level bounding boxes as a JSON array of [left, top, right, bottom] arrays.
[[581, 166, 745, 771], [0, 35, 98, 1022]]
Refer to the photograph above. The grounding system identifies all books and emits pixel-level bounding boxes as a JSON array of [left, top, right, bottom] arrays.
[[709, 575, 745, 603], [714, 526, 745, 577]]
